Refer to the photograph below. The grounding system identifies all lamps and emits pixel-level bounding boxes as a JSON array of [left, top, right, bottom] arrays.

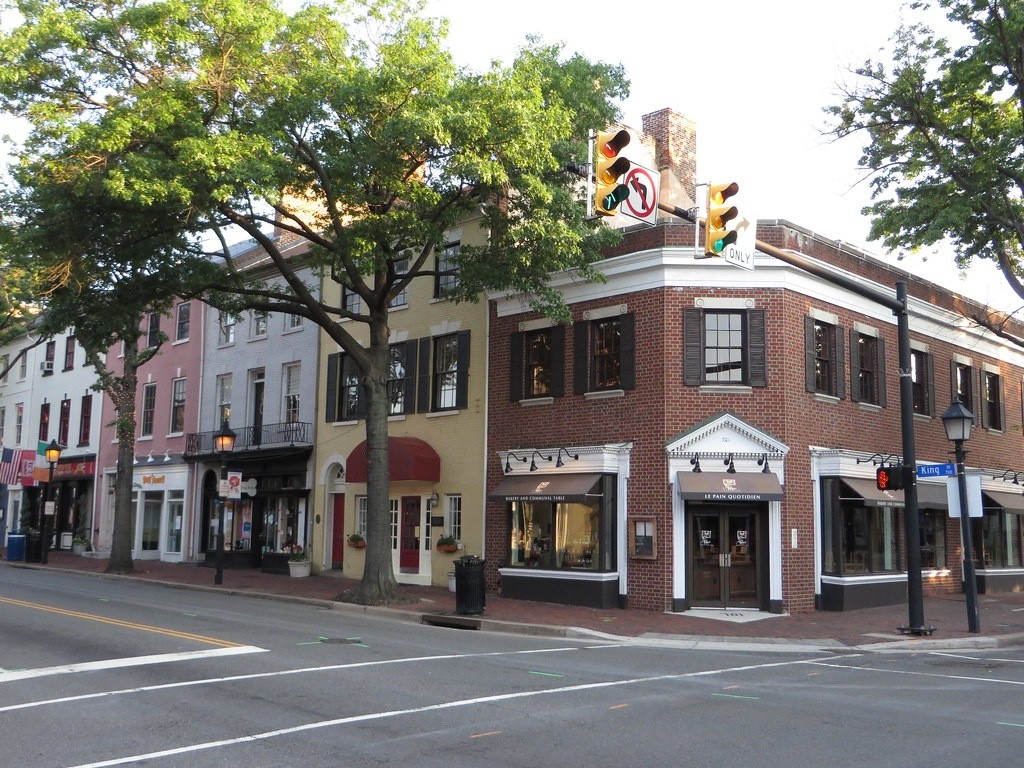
[[134, 449, 175, 465], [993, 469, 1024, 487], [857, 453, 902, 469], [429, 491, 439, 507], [724, 452, 736, 474], [758, 453, 772, 474], [556, 446, 579, 467], [505, 453, 527, 474], [529, 451, 552, 471], [690, 452, 702, 474]]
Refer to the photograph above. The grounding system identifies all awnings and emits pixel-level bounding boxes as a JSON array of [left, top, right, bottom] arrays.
[[345, 437, 441, 486], [677, 471, 784, 501], [489, 473, 603, 502], [839, 477, 948, 510], [983, 490, 1024, 514]]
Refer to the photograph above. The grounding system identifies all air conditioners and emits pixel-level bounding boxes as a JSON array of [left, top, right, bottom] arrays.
[[41, 361, 53, 371]]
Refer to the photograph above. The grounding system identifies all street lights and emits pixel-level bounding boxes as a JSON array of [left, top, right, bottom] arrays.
[[939, 396, 982, 632], [213, 418, 238, 584], [41, 438, 62, 566]]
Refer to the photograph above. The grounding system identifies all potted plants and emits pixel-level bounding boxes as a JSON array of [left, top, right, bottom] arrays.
[[448, 571, 457, 592], [436, 536, 457, 552], [288, 553, 313, 578], [73, 537, 89, 556], [348, 534, 365, 547]]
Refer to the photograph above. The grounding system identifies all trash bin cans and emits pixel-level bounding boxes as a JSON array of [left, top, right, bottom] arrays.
[[25, 528, 42, 562], [452, 555, 485, 614], [7, 529, 25, 561]]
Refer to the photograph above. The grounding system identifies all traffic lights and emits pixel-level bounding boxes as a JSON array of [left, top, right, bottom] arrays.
[[704, 181, 739, 258], [876, 464, 913, 491], [594, 129, 631, 217]]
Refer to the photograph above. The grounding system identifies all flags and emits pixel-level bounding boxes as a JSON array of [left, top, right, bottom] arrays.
[[0, 448, 22, 485], [21, 450, 39, 486], [32, 440, 63, 482]]
